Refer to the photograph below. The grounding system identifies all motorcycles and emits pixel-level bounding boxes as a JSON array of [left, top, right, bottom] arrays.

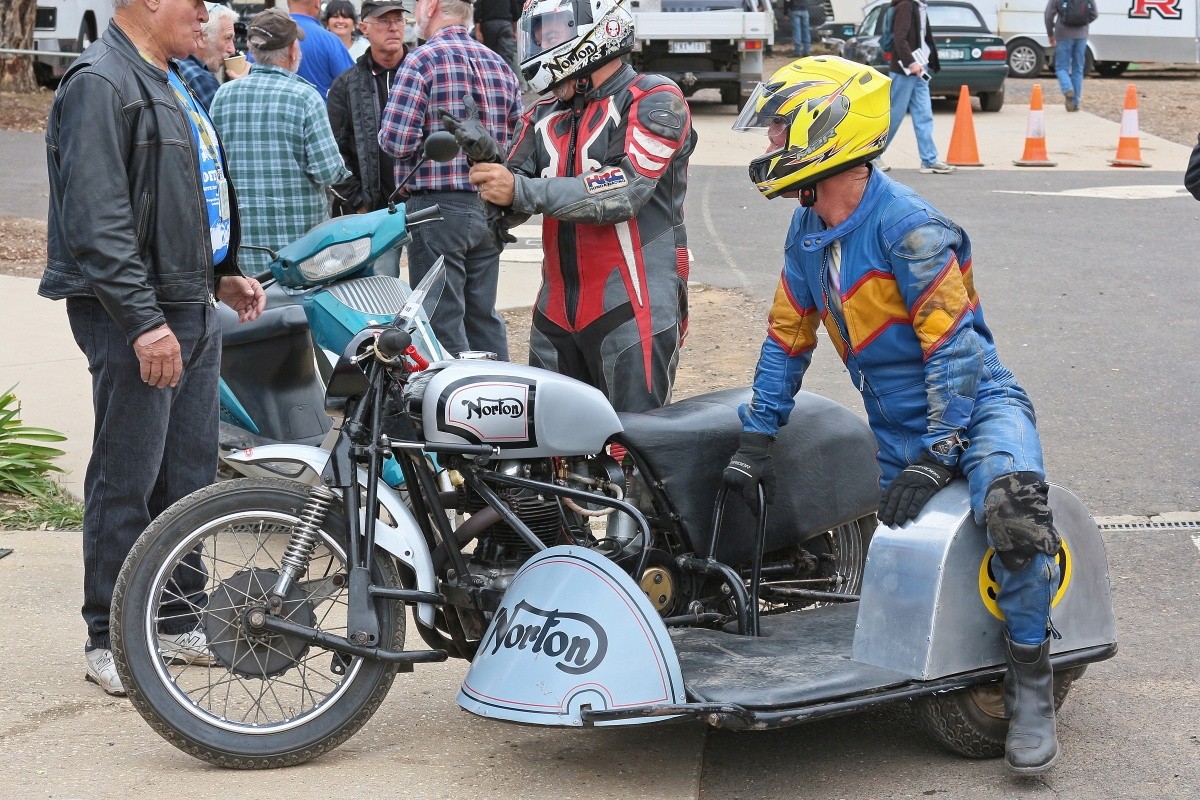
[[105, 250, 1123, 773]]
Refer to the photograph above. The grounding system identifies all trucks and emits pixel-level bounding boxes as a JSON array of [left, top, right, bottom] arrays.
[[994, 0, 1200, 80]]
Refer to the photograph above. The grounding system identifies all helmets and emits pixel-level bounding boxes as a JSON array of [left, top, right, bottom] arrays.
[[517, 0, 635, 96], [748, 55, 892, 200]]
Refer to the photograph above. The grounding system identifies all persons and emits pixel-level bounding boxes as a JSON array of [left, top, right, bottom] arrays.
[[326, 0, 416, 278], [436, 0, 698, 410], [875, 0, 957, 174], [247, 0, 354, 105], [175, 2, 252, 110], [722, 55, 1060, 778], [209, 7, 360, 276], [39, 0, 266, 696], [376, 0, 525, 358], [783, 0, 811, 58], [1044, 0, 1098, 112], [473, 0, 516, 62], [322, 0, 372, 63]]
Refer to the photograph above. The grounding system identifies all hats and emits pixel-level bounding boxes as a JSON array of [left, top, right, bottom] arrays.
[[361, 0, 412, 22], [246, 8, 304, 49]]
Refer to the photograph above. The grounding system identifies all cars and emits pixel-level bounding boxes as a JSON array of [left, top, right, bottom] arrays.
[[842, 0, 1010, 113], [29, 1, 125, 88], [603, 1, 776, 119]]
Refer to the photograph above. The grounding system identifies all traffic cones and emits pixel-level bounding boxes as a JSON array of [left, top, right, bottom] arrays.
[[1105, 82, 1153, 169], [1012, 83, 1060, 168], [940, 84, 986, 168]]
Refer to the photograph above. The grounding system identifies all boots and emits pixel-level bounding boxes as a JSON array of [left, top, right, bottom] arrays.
[[1003, 626, 1060, 773]]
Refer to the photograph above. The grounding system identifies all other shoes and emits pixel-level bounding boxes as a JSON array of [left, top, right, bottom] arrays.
[[1065, 89, 1075, 111]]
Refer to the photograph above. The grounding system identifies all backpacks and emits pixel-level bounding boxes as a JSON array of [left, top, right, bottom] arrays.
[[880, 2, 897, 50], [1061, 0, 1092, 28]]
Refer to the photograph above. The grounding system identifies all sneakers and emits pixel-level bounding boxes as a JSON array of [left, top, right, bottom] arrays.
[[870, 159, 891, 173], [156, 631, 223, 667], [84, 637, 128, 695], [919, 159, 957, 174]]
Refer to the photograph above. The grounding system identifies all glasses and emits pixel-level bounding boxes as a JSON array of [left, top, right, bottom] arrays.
[[364, 17, 408, 27]]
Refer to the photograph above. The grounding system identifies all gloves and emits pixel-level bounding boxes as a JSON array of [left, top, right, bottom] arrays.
[[723, 432, 775, 515], [877, 452, 953, 526], [437, 95, 506, 167], [486, 200, 517, 243]]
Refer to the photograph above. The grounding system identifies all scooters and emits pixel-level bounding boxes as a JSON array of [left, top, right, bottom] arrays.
[[208, 133, 482, 599]]
